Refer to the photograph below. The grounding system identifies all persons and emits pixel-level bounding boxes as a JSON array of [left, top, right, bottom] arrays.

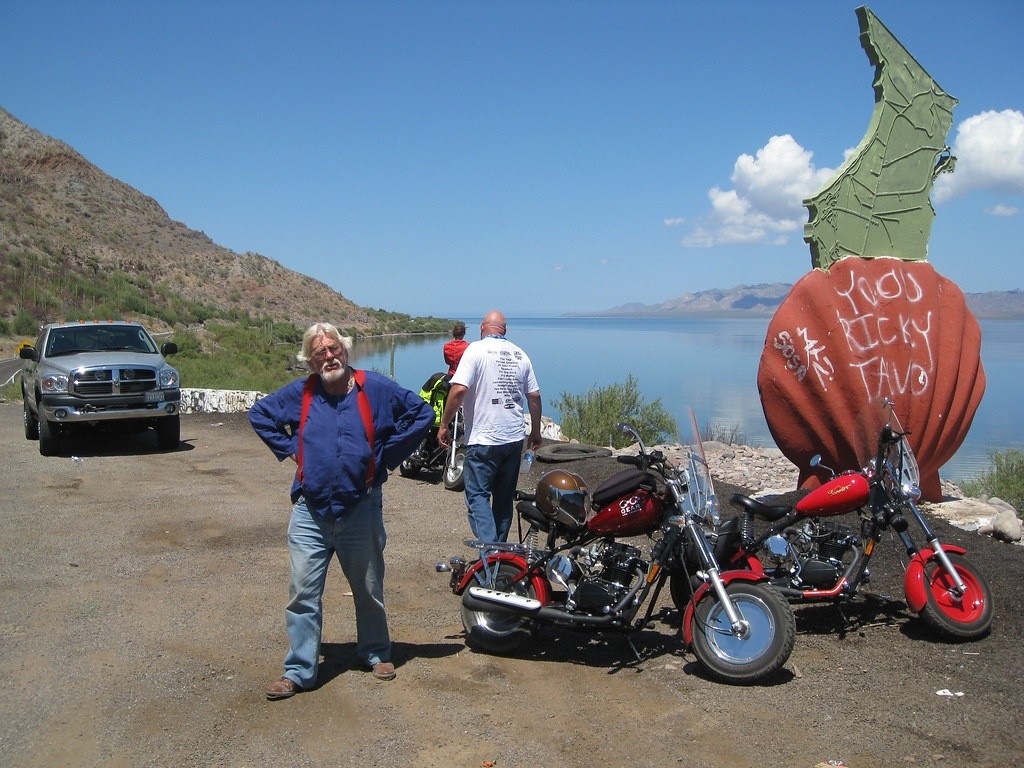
[[248, 322, 436, 698], [444, 325, 469, 439], [437, 311, 543, 560]]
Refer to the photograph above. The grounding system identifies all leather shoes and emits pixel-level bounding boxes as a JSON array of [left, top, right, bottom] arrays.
[[372, 660, 396, 681], [266, 677, 300, 698]]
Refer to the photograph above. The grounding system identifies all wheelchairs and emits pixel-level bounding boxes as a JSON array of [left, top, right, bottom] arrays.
[[399, 404, 466, 493]]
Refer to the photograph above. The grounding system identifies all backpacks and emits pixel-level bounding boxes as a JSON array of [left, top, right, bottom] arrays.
[[418, 372, 454, 429]]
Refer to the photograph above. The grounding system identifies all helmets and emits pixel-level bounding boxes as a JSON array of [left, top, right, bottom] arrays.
[[535, 469, 592, 526]]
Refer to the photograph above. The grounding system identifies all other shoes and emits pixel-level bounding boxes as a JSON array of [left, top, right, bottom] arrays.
[[464, 540, 482, 548]]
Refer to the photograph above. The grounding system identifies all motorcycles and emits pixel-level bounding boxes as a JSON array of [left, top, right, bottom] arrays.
[[434, 423, 797, 687], [668, 395, 995, 642]]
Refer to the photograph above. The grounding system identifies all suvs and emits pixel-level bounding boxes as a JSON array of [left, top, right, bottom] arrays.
[[19, 319, 182, 458]]
